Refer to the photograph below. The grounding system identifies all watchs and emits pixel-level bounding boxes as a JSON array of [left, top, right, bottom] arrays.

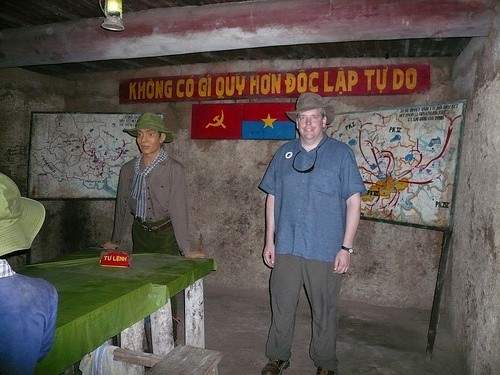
[[341, 245, 354, 254]]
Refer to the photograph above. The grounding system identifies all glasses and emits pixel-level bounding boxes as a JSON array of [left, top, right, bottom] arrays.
[[291, 150, 318, 173]]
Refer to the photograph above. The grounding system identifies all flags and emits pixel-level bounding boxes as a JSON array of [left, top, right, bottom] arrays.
[[191, 103, 241, 139], [241, 102, 297, 140]]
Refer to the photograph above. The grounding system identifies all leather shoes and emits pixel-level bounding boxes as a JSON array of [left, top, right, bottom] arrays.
[[312, 367, 339, 375], [259, 354, 293, 375]]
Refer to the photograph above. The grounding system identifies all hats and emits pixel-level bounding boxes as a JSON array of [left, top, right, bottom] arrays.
[[0, 171, 47, 257], [120, 113, 173, 145], [284, 92, 336, 127]]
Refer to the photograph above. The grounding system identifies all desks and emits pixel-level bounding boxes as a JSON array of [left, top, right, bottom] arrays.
[[16, 248, 218, 375]]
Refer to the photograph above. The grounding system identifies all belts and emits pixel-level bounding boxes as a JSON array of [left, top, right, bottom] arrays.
[[134, 221, 169, 232]]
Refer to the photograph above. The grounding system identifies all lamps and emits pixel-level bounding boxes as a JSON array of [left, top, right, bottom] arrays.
[[99, 0, 125, 31]]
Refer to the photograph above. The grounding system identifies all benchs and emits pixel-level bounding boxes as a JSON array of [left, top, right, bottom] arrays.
[[113, 344, 224, 375]]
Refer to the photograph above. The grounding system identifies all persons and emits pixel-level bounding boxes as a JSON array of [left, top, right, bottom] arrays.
[[0, 173, 58, 375], [97, 112, 206, 353], [258, 93, 368, 375]]
